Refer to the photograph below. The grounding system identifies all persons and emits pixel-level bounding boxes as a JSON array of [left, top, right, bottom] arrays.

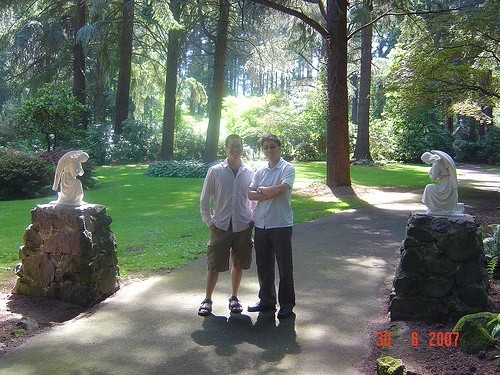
[[247, 133, 296, 319], [421, 150, 459, 215], [198, 134, 256, 316], [51, 150, 89, 206]]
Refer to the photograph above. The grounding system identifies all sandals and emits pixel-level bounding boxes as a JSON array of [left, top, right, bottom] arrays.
[[228, 296, 243, 313], [198, 298, 212, 316]]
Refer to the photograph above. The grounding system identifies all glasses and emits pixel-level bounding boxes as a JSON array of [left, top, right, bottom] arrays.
[[262, 145, 280, 150]]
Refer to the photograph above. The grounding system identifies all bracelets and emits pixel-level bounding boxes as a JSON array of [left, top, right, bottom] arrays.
[[259, 187, 262, 194]]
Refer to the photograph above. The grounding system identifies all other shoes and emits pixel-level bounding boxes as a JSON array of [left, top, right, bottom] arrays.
[[248, 301, 275, 312], [277, 304, 294, 318]]
